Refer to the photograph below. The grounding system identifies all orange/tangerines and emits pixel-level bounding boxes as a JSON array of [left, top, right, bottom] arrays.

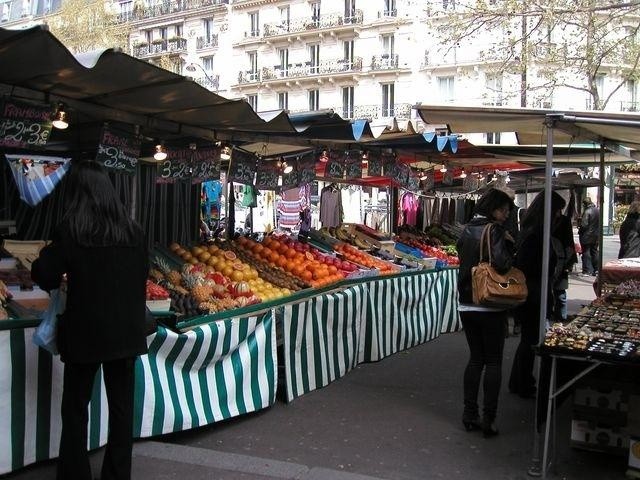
[[235, 235, 398, 289]]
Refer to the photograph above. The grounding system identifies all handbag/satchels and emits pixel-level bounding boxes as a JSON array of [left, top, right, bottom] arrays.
[[472, 223, 527, 307], [32, 272, 69, 355]]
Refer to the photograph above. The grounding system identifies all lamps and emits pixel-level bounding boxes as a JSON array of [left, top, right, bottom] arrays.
[[153, 137, 167, 161], [318, 149, 328, 162], [280, 157, 293, 173], [220, 143, 232, 160], [52, 101, 69, 130]]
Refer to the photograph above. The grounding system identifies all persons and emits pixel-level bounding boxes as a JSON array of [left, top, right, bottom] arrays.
[[578, 196, 599, 276], [618, 201, 640, 258], [455, 189, 515, 438], [32, 160, 148, 478], [510, 191, 565, 399], [551, 211, 577, 323]]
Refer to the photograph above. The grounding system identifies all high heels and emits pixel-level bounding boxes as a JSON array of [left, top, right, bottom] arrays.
[[508, 389, 535, 400], [462, 418, 482, 432], [482, 424, 500, 438]]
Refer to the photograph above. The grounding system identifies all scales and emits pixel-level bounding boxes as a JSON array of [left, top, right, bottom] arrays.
[[3, 239, 45, 270]]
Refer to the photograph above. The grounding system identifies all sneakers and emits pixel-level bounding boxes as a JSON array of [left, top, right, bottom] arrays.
[[577, 270, 599, 277], [530, 376, 535, 384]]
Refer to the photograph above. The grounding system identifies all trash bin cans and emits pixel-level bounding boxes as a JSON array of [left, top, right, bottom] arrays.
[[603, 226, 615, 236]]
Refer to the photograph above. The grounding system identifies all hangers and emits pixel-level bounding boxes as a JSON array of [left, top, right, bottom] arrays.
[[326, 181, 339, 193], [404, 185, 482, 202]]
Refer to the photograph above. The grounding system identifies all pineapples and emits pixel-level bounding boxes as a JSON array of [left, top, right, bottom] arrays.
[[141, 255, 238, 314]]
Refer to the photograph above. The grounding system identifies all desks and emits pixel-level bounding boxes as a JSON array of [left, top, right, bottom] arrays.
[[529, 301, 640, 480]]
